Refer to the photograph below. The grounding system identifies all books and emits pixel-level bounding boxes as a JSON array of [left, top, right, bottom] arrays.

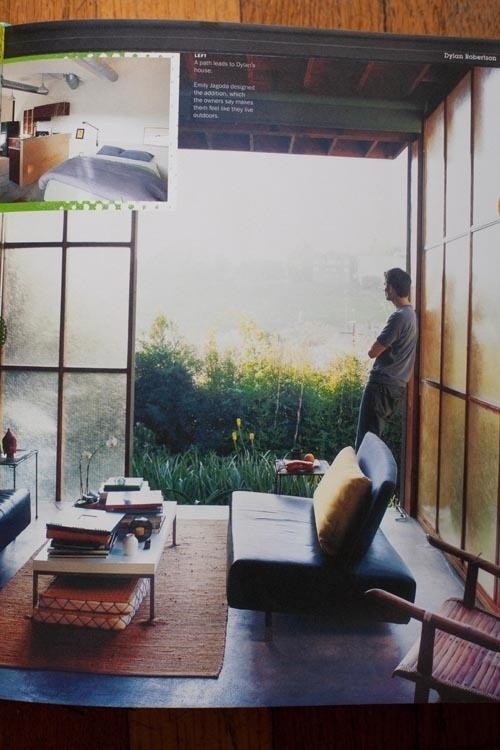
[[44, 475, 167, 559]]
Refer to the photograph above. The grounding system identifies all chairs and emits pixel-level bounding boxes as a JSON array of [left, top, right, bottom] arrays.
[[361, 532, 498, 704]]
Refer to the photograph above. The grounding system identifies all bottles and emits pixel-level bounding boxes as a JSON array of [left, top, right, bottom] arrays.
[[123, 533, 139, 556]]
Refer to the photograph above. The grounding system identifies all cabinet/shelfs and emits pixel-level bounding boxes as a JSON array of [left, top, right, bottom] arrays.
[[0, 450, 39, 520]]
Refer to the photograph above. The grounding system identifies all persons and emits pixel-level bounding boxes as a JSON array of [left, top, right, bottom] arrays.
[[353, 266, 419, 458]]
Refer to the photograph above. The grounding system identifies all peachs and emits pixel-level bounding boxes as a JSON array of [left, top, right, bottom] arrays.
[[304, 453, 314, 462]]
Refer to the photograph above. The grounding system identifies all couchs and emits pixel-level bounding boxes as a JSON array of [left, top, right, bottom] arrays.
[[0, 486, 32, 551], [227, 431, 418, 640]]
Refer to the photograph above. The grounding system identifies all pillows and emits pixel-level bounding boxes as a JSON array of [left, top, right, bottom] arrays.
[[310, 445, 374, 554]]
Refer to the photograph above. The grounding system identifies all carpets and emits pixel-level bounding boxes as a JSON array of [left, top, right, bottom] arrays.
[[0, 516, 231, 678]]
